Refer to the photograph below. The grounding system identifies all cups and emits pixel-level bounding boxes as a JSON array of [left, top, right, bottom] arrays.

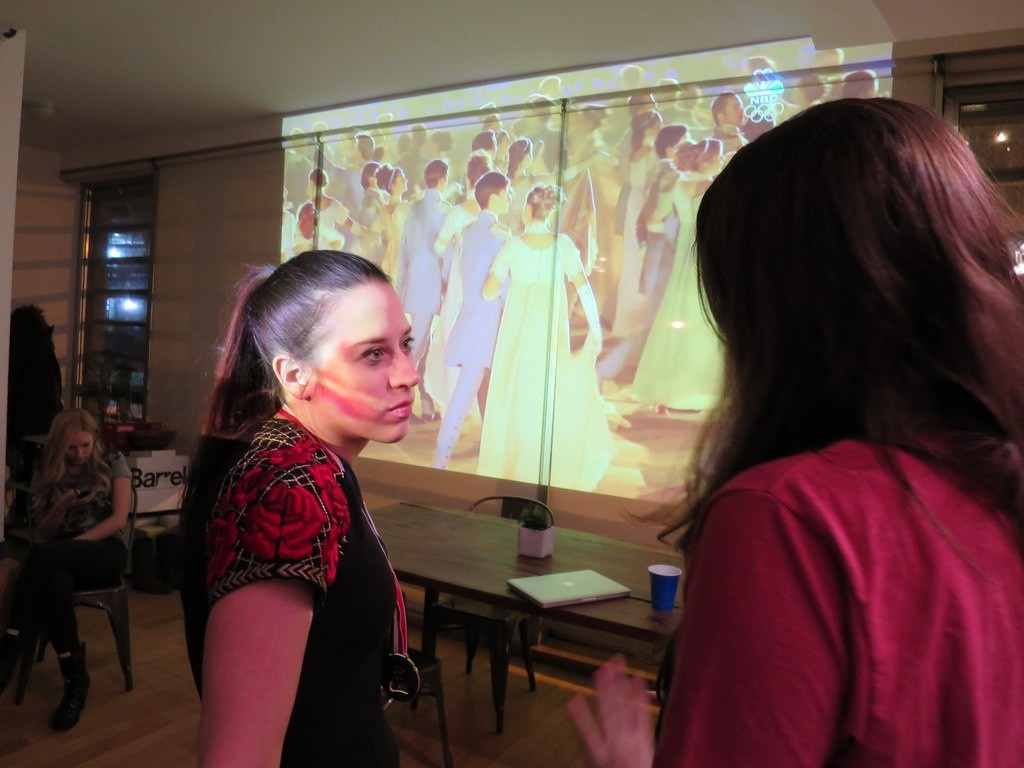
[[648, 564, 683, 612]]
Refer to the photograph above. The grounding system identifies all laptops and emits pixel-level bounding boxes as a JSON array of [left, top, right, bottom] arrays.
[[506, 568, 631, 610]]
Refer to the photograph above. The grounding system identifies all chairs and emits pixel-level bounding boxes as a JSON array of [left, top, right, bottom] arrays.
[[410, 495, 558, 738], [9, 483, 140, 708]]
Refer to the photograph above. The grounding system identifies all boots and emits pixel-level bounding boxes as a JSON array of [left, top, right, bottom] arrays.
[[51, 642, 90, 730], [0, 632, 20, 695]]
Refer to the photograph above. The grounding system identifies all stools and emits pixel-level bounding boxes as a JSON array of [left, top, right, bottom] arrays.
[[138, 524, 180, 579], [403, 646, 452, 768]]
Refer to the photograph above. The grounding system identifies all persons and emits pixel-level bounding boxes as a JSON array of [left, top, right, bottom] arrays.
[[566, 98, 1024, 768], [177, 250, 420, 768], [282, 45, 879, 494], [0, 407, 133, 731]]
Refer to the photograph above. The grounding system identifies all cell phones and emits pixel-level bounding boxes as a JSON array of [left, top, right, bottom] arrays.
[[77, 490, 91, 499]]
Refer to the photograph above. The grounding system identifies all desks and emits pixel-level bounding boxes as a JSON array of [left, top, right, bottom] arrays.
[[350, 502, 689, 734]]
